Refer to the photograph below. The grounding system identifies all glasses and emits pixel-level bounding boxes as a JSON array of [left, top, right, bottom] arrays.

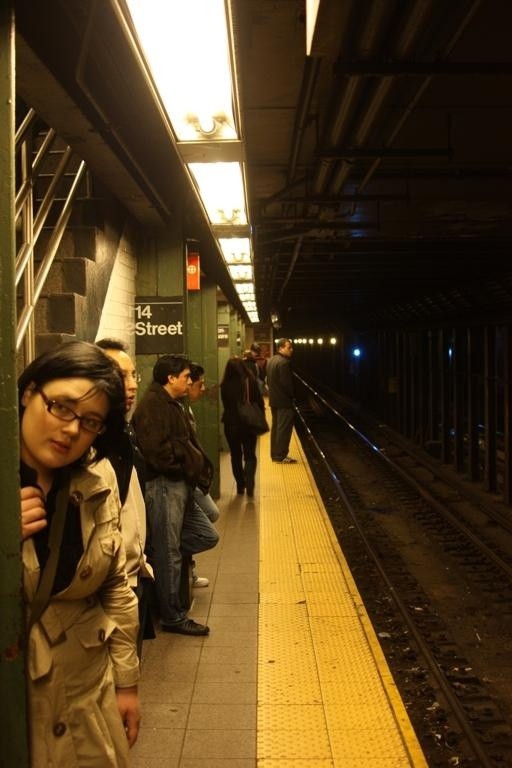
[[38, 387, 105, 435], [120, 371, 142, 382]]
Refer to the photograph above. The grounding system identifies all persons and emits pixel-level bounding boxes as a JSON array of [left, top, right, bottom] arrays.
[[241, 349, 259, 377], [219, 355, 265, 497], [15, 338, 145, 767], [95, 337, 159, 642], [174, 360, 219, 586], [128, 351, 219, 638], [250, 341, 269, 390], [265, 337, 299, 464]]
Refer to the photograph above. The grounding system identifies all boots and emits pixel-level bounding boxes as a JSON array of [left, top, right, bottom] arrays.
[[236, 487, 254, 496], [160, 620, 209, 636]]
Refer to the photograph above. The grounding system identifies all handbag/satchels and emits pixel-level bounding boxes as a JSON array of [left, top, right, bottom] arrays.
[[236, 400, 270, 436]]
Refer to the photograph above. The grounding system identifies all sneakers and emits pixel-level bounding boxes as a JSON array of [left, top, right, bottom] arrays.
[[192, 577, 210, 587], [272, 457, 297, 464]]
